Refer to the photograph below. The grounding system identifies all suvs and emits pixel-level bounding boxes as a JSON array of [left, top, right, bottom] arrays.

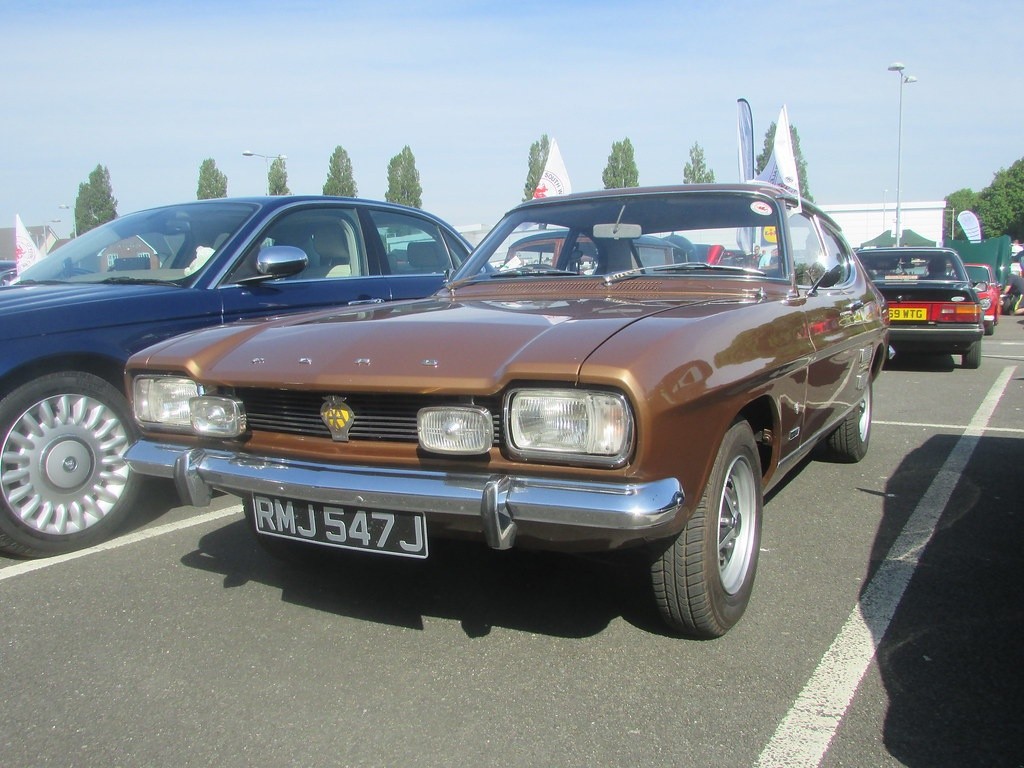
[[497, 229, 689, 278], [0, 196, 499, 561]]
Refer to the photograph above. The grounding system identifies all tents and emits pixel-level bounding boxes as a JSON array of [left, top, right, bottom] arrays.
[[861, 229, 936, 248], [942, 235, 1013, 291]]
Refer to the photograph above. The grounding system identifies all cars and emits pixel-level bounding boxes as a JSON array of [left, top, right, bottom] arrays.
[[851, 246, 988, 369], [123, 179, 895, 639], [941, 264, 1003, 335]]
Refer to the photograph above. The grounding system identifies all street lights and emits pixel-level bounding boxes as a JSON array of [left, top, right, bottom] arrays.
[[58, 203, 78, 239], [43, 218, 61, 255], [242, 151, 288, 196]]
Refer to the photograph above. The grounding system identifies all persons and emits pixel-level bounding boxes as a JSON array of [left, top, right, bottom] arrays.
[[1000, 271, 1024, 316], [2, 275, 9, 286], [916, 258, 958, 281], [752, 244, 779, 271]]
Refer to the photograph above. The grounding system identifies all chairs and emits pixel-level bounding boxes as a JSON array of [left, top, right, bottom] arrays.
[[868, 257, 898, 279], [926, 258, 948, 279], [214, 224, 445, 280]]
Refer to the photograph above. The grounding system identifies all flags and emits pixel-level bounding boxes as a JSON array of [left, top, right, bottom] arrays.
[[14, 214, 42, 277], [520, 140, 571, 231], [752, 105, 802, 219]]
